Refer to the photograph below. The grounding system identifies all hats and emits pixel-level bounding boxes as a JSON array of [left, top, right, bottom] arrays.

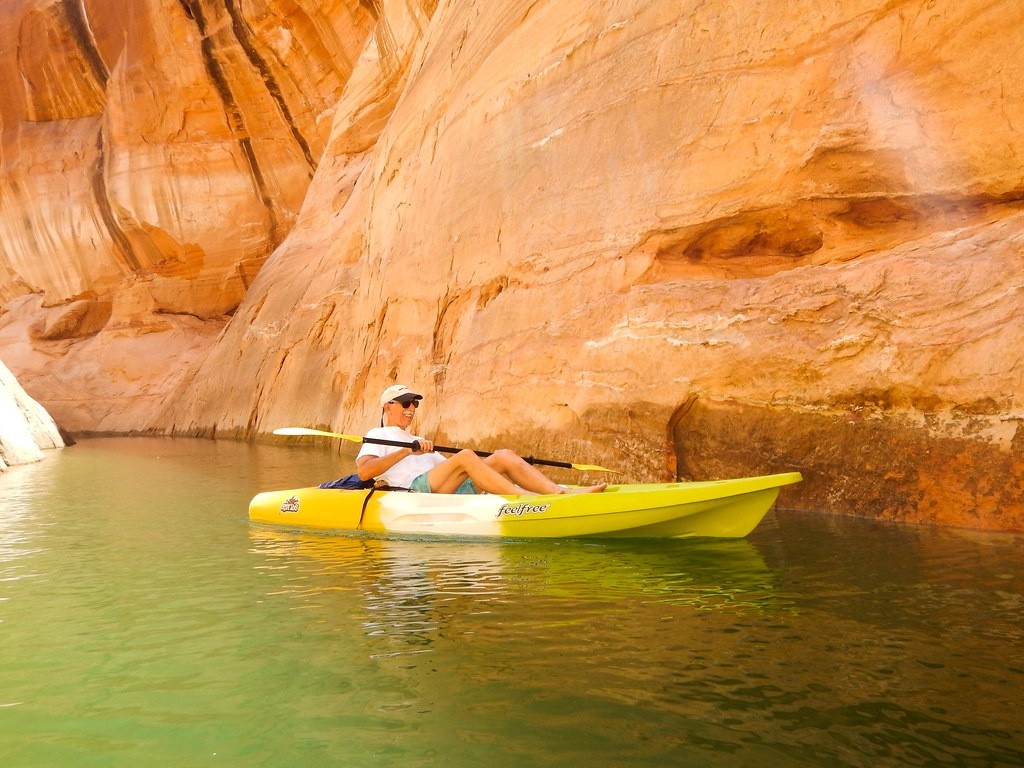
[[380, 385, 423, 407]]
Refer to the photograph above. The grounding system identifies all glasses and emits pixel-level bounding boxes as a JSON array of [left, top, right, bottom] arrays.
[[389, 400, 420, 408]]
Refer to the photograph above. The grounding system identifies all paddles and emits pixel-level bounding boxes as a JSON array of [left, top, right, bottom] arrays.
[[272, 427, 625, 477]]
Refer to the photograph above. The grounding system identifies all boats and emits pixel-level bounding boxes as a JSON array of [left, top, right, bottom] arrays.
[[246, 469, 807, 538]]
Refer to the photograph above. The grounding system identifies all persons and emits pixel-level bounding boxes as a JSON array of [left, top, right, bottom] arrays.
[[355, 385, 607, 495]]
